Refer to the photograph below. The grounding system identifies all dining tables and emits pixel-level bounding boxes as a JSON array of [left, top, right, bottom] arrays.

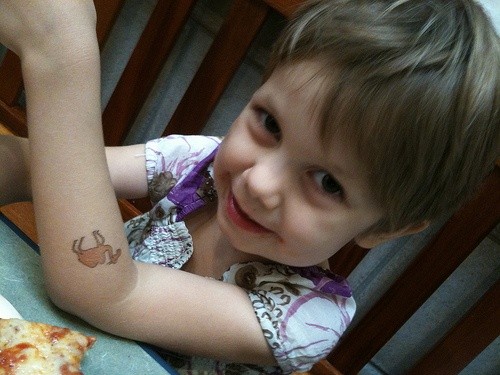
[[0, 212, 176, 375]]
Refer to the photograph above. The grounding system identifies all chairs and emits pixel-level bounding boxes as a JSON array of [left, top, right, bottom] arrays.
[[0, 0, 500, 375]]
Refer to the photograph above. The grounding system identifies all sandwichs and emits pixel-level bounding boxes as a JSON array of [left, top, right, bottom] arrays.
[[0, 318, 95, 375]]
